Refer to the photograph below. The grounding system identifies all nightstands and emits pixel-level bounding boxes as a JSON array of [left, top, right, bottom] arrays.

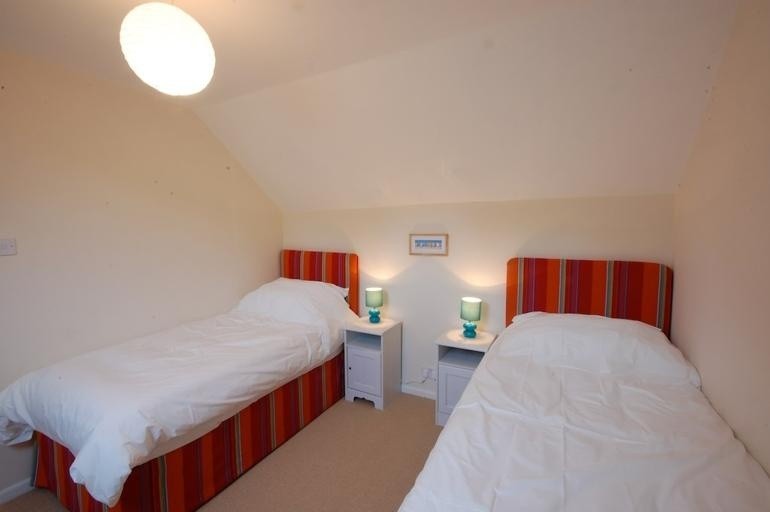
[[344, 314, 401, 410], [434, 328, 496, 428]]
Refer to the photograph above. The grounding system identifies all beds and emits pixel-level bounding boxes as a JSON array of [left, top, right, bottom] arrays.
[[35, 252, 359, 511], [399, 258, 769, 510]]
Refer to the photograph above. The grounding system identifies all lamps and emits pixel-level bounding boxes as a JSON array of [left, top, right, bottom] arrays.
[[462, 297, 480, 339], [365, 288, 384, 323]]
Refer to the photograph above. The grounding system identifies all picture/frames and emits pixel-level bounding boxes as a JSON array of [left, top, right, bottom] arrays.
[[408, 235, 447, 255]]
